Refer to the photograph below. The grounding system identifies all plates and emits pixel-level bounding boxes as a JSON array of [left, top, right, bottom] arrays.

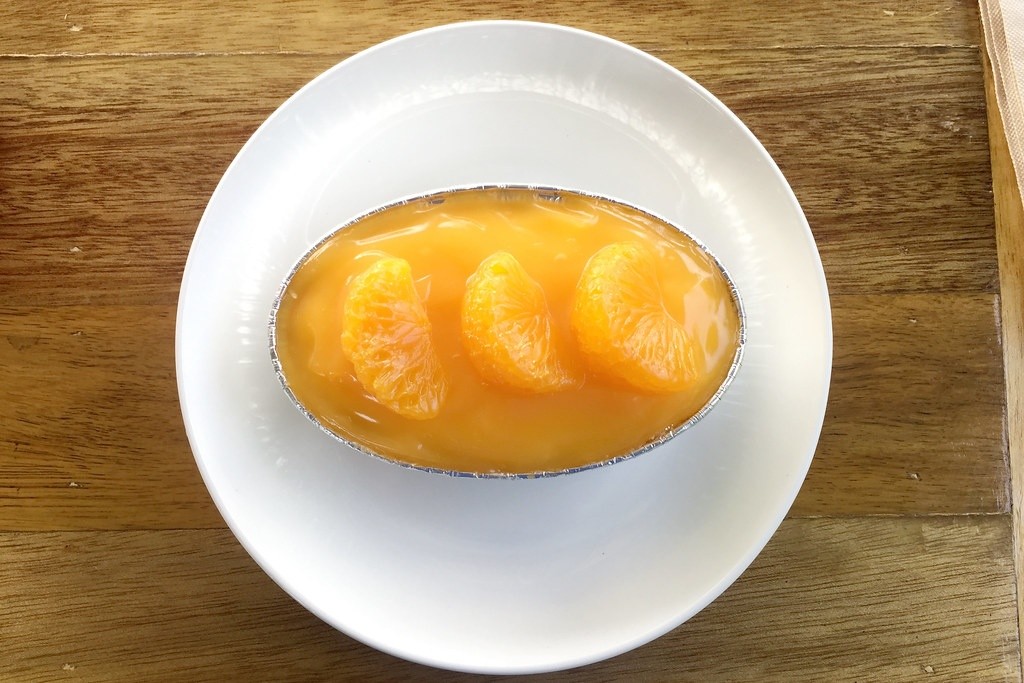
[[174, 20, 833, 673]]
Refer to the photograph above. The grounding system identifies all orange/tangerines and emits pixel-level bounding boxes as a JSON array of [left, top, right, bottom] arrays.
[[343, 259, 447, 420], [574, 243, 698, 388], [462, 254, 572, 389]]
